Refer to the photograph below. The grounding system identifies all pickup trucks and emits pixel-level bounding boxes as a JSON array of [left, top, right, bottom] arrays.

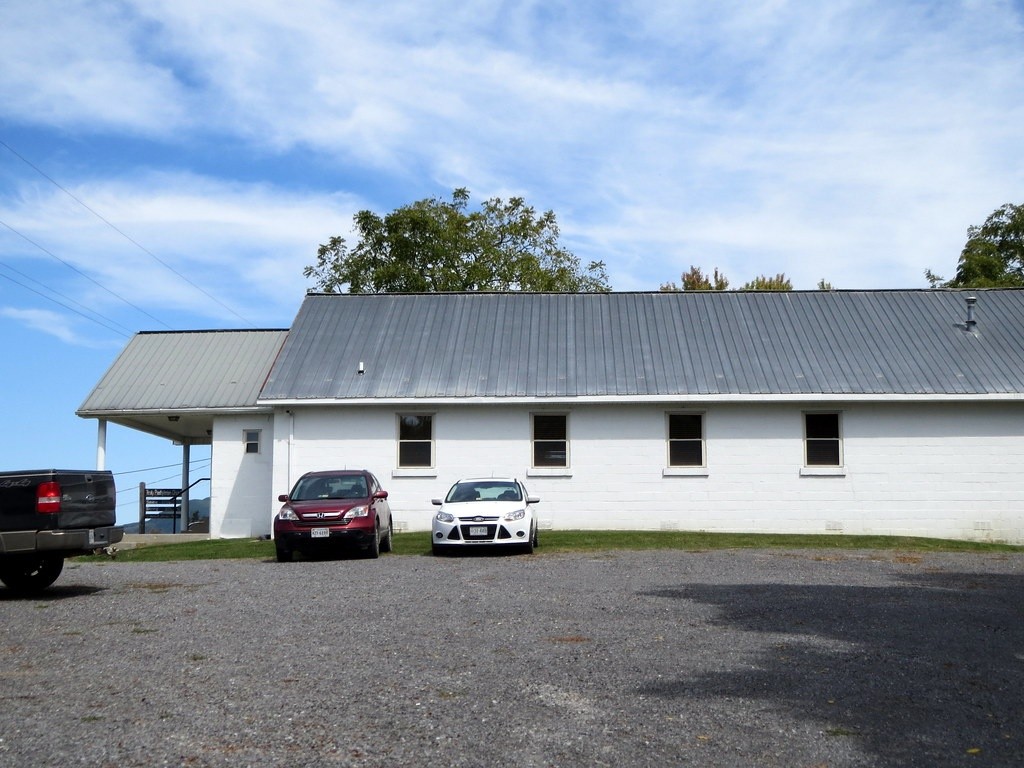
[[0, 468, 126, 590]]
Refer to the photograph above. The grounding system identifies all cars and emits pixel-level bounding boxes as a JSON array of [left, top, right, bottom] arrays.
[[431, 477, 541, 553], [272, 469, 393, 561]]
